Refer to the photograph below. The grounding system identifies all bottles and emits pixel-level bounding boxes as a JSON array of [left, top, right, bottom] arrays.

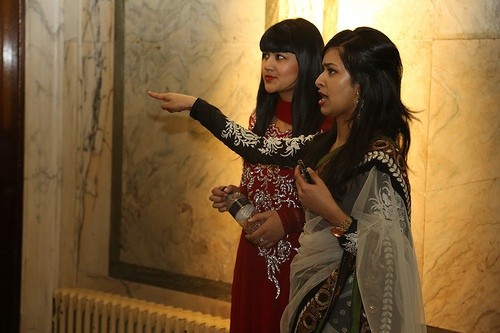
[[221, 186, 264, 234]]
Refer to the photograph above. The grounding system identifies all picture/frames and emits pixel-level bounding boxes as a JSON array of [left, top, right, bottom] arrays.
[[108, 1, 278, 304]]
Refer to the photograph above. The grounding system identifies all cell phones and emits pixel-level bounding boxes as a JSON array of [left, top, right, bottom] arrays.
[[298, 160, 313, 185]]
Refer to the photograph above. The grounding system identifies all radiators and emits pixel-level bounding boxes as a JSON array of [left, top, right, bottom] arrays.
[[53, 287, 230, 333]]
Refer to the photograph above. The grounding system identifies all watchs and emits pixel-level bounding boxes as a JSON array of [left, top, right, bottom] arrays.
[[331, 214, 353, 238]]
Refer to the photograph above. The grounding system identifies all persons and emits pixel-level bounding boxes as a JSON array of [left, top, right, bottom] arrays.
[[148, 27, 428, 333], [210, 18, 337, 332]]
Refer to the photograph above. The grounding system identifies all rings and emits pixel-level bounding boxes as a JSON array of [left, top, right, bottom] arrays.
[[259, 237, 264, 243]]
